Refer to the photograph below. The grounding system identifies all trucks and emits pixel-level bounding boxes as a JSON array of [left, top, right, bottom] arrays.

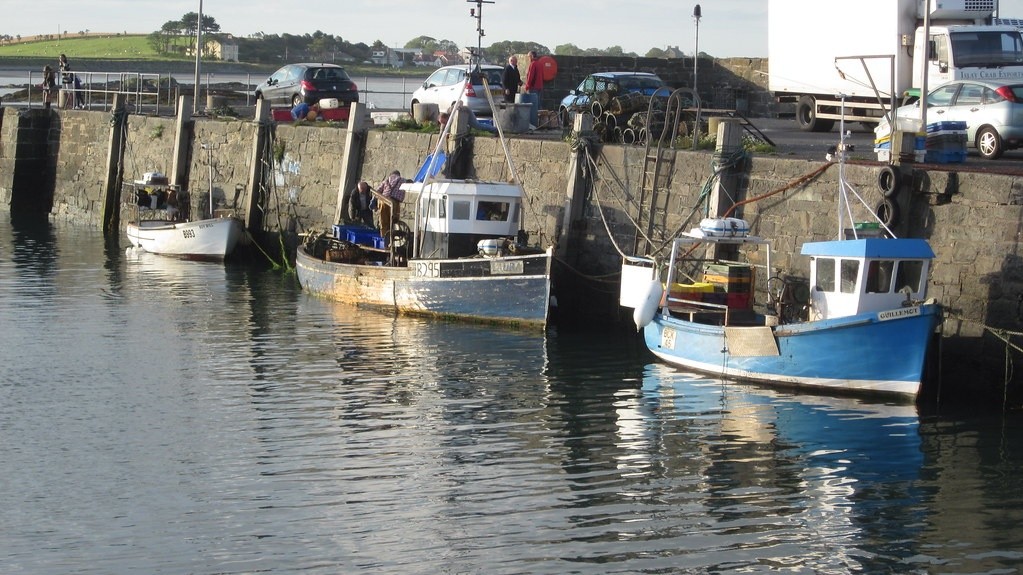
[[766, 0, 1023, 134]]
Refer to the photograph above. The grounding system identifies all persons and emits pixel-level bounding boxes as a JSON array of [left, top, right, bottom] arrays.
[[347, 182, 374, 228], [430, 105, 500, 155], [523, 50, 543, 130], [59, 53, 86, 109], [42, 65, 55, 107], [501, 56, 523, 103], [367, 170, 413, 237]]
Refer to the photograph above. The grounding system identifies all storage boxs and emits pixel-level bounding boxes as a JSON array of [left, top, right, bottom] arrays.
[[332, 224, 374, 241], [703, 263, 751, 308], [662, 292, 729, 310], [346, 229, 381, 244], [873, 117, 969, 163], [372, 236, 386, 249], [844, 228, 885, 240], [854, 222, 879, 230], [272, 109, 292, 121], [320, 106, 349, 121]]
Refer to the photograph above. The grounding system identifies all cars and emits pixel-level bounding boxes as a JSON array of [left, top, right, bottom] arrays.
[[875, 77, 1023, 160], [408, 63, 506, 125], [253, 63, 359, 110], [558, 71, 671, 129]]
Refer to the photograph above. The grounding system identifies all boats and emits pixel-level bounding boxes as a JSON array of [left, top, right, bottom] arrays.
[[290, 0, 587, 336], [618, 53, 948, 406], [122, 128, 247, 268]]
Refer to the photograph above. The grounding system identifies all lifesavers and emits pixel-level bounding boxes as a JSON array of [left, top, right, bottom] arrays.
[[876, 165, 901, 196], [873, 196, 901, 230]]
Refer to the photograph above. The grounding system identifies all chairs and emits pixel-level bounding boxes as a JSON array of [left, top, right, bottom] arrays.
[[489, 74, 501, 85], [969, 90, 982, 97]]
